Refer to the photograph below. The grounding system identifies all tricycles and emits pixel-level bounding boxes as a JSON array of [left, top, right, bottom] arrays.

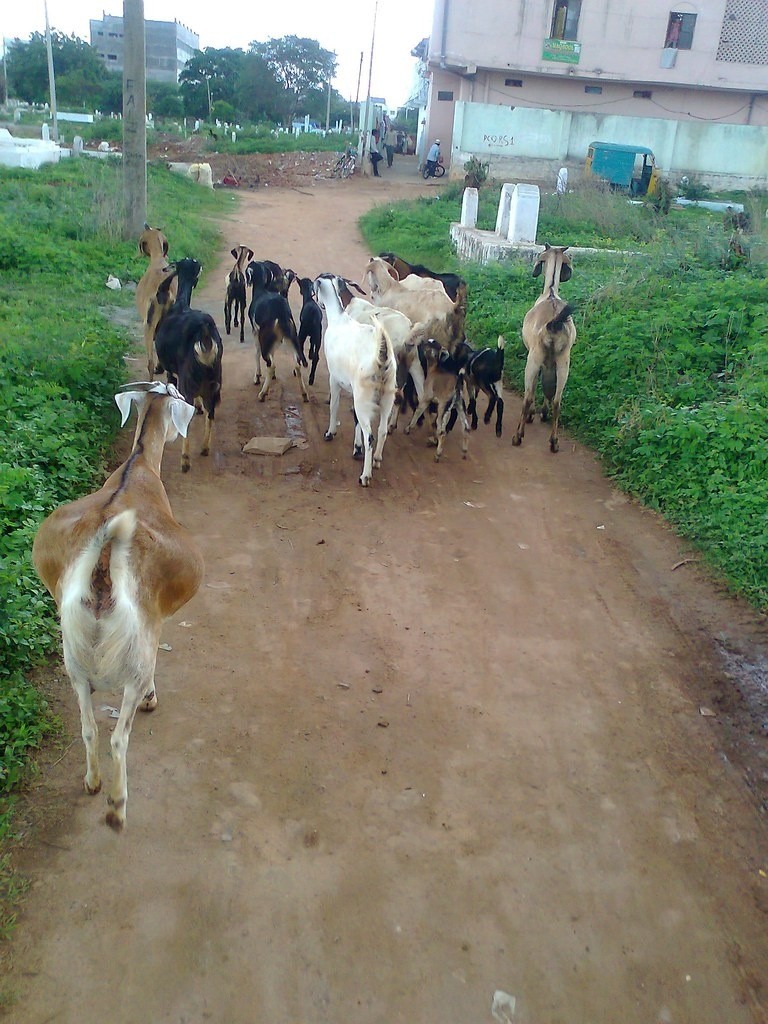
[[584, 141, 662, 201]]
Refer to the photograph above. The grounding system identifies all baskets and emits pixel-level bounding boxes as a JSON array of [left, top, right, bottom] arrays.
[[439, 156, 443, 163]]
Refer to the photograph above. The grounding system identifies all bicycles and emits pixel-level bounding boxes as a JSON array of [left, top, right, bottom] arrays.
[[330, 141, 358, 180], [422, 153, 446, 179]]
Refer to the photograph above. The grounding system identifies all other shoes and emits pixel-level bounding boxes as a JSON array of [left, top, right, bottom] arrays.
[[370, 159, 375, 165], [375, 175, 381, 177], [387, 164, 392, 168]]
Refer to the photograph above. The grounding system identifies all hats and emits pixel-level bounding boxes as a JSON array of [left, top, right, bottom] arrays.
[[436, 139, 440, 144]]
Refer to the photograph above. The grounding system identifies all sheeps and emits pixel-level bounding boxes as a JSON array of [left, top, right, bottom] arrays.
[[33, 380, 204, 833], [135, 222, 222, 473], [224, 244, 322, 404], [313, 254, 505, 486], [512, 243, 575, 452]]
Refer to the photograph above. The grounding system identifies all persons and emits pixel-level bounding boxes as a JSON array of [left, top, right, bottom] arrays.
[[369, 129, 383, 177], [385, 125, 397, 168], [666, 18, 681, 49], [427, 139, 441, 178], [396, 131, 406, 152]]
